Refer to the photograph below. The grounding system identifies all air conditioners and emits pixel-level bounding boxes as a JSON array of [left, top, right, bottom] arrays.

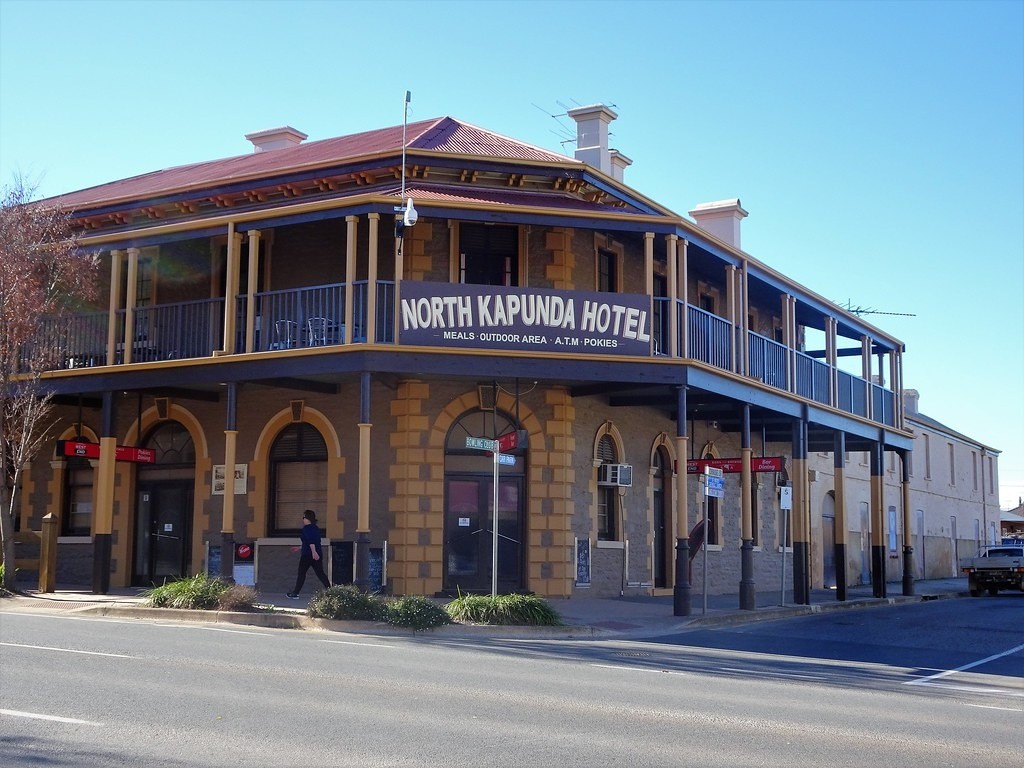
[[596, 462, 632, 487]]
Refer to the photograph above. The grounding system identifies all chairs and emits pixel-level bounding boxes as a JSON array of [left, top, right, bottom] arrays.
[[274, 316, 334, 348]]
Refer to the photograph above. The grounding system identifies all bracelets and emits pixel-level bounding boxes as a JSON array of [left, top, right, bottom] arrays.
[[314, 553, 316, 555]]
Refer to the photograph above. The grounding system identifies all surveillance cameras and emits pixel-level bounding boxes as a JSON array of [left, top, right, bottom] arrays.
[[406, 209, 418, 224]]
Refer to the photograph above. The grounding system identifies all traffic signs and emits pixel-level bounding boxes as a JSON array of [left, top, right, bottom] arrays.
[[707, 467, 725, 498], [466, 437, 516, 467]]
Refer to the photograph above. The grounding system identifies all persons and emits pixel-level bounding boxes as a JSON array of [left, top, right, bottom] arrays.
[[285, 510, 331, 599]]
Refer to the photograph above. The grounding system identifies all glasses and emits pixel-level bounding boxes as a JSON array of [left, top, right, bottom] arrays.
[[303, 517, 306, 519]]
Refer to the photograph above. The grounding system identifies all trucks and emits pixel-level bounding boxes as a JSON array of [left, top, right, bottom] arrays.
[[960, 544, 1024, 597]]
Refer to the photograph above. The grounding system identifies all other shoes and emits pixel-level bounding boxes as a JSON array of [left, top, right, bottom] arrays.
[[286, 592, 300, 599]]
